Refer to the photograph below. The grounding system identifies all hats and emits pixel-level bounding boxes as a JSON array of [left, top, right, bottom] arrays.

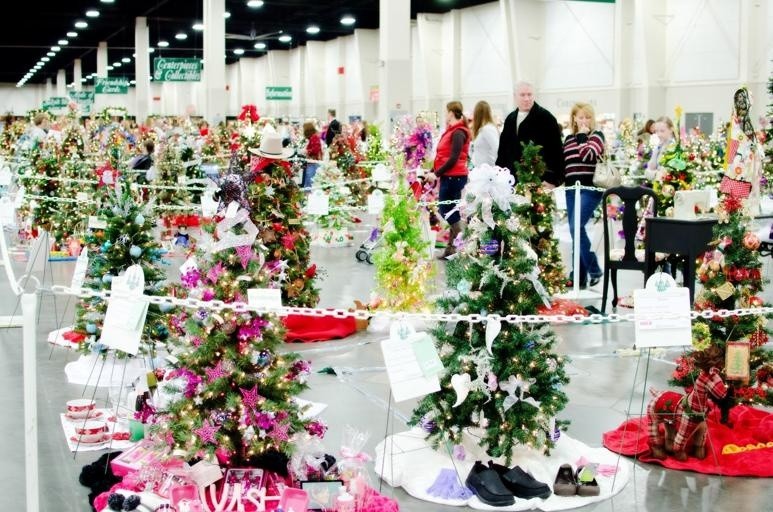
[[245, 131, 297, 161]]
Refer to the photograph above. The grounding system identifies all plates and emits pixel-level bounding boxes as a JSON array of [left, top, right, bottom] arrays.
[[71, 437, 110, 446], [63, 412, 103, 420]]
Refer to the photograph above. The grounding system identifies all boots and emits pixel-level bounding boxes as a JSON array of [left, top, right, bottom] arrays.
[[438, 220, 471, 261]]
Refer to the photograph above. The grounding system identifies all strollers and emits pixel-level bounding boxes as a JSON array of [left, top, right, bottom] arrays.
[[352, 175, 441, 265], [756, 221, 773, 259]]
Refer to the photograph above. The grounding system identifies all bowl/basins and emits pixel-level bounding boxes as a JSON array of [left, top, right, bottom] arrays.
[[64, 399, 94, 417], [75, 421, 105, 441]]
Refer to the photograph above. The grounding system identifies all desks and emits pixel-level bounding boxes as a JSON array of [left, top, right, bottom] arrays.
[[641, 213, 720, 310]]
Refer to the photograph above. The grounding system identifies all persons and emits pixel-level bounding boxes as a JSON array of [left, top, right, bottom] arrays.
[[495, 82, 566, 190], [423, 101, 472, 260], [647, 117, 677, 170], [562, 102, 605, 286], [471, 101, 501, 167], [637, 120, 655, 144]]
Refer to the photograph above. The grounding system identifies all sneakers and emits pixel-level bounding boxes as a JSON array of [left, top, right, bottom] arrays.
[[574, 464, 602, 498], [551, 462, 578, 497]]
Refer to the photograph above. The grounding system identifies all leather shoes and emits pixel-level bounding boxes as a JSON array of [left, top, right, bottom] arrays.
[[565, 273, 603, 289], [487, 459, 553, 500], [464, 459, 516, 508]]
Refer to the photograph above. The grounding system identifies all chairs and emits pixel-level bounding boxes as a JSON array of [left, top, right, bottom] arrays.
[[596, 183, 675, 314]]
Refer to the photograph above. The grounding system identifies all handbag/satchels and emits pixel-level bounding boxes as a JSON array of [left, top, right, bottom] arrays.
[[590, 136, 623, 190]]
